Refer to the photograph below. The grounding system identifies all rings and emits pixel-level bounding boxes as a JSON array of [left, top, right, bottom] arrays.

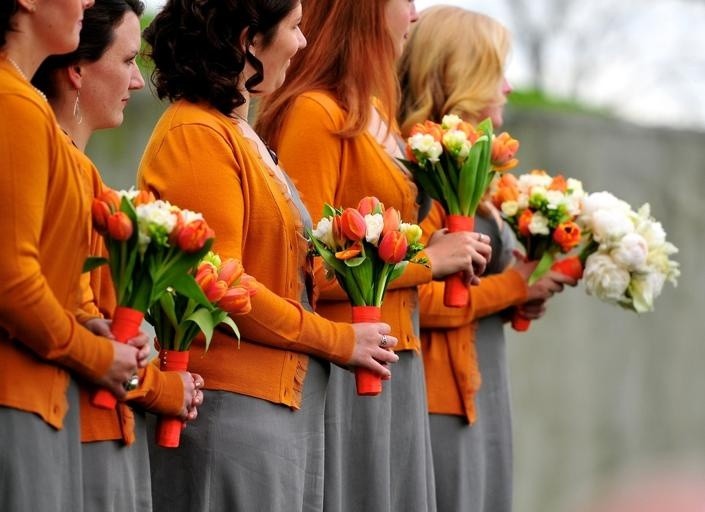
[[381, 336, 386, 347], [480, 232, 484, 241], [127, 375, 140, 390]]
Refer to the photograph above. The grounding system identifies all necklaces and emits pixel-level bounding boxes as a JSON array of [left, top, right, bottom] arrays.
[[7, 55, 49, 104], [234, 111, 278, 166]]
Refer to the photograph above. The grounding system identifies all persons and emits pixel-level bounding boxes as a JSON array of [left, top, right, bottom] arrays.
[[1, 2, 151, 512], [399, 5, 579, 512], [255, 1, 493, 512], [41, 0, 207, 512], [138, 0, 399, 512]]
